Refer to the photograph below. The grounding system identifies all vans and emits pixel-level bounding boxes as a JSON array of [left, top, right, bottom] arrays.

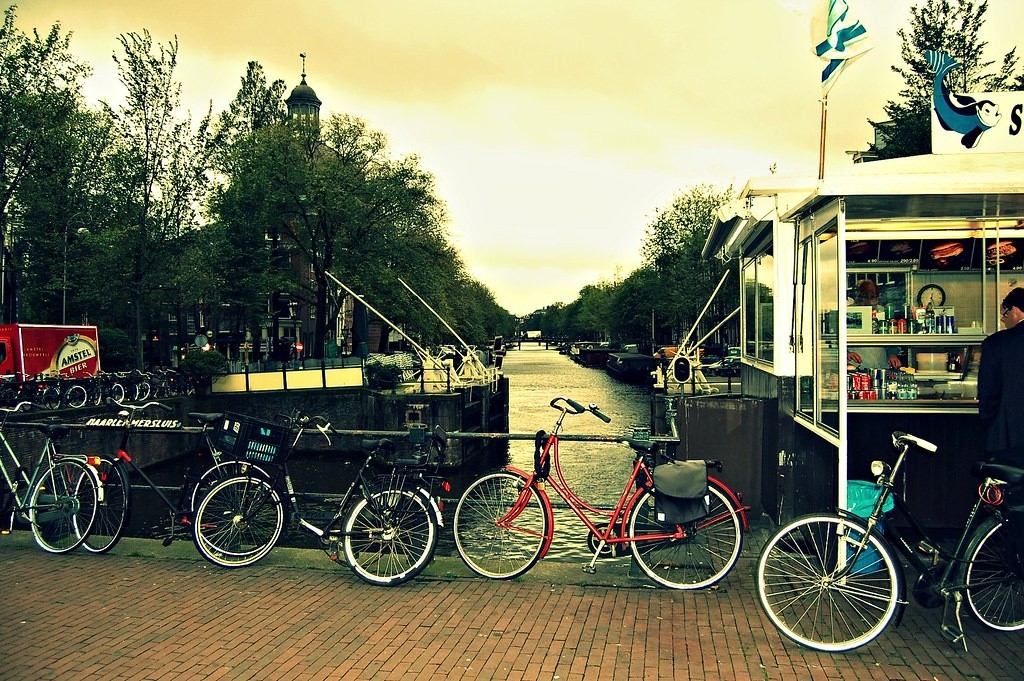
[[654, 347, 704, 361], [623, 344, 638, 354]]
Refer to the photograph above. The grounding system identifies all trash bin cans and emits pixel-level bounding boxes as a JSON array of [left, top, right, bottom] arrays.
[[847, 479, 894, 574]]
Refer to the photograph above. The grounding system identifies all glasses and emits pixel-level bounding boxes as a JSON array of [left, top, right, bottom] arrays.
[[1003, 308, 1011, 318]]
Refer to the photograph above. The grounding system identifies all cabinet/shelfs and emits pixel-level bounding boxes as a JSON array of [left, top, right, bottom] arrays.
[[818, 331, 988, 528]]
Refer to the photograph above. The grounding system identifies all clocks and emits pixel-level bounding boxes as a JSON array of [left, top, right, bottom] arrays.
[[916, 284, 946, 307]]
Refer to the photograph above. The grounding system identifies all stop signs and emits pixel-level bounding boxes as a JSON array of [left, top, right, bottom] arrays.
[[295, 342, 304, 352]]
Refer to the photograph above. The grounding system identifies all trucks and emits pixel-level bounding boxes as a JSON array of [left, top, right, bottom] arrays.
[[0, 324, 102, 383]]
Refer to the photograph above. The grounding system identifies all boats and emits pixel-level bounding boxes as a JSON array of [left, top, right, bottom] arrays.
[[557, 340, 661, 385]]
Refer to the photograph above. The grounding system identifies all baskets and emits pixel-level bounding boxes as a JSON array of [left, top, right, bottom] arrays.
[[215, 411, 292, 465]]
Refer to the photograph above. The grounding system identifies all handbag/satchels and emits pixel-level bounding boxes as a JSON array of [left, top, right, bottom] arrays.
[[653, 459, 710, 524]]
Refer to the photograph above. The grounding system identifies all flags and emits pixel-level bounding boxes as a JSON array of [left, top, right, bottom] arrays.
[[811, 0, 873, 99]]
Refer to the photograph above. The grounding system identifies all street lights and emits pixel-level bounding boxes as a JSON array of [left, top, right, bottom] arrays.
[[61, 210, 94, 323]]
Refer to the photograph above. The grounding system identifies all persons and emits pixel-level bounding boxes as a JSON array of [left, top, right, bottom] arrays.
[[0, 351, 4, 362], [978, 287, 1024, 591], [290, 339, 299, 361], [453, 346, 474, 368], [344, 326, 350, 345], [327, 332, 330, 341], [830, 280, 901, 369]]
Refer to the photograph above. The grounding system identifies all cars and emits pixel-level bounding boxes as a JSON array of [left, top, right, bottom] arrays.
[[707, 357, 742, 376], [435, 344, 456, 355], [706, 347, 741, 361]]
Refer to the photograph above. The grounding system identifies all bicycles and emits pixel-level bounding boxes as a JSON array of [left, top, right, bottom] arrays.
[[0, 368, 196, 427], [0, 401, 748, 594], [755, 430, 1024, 651]]
